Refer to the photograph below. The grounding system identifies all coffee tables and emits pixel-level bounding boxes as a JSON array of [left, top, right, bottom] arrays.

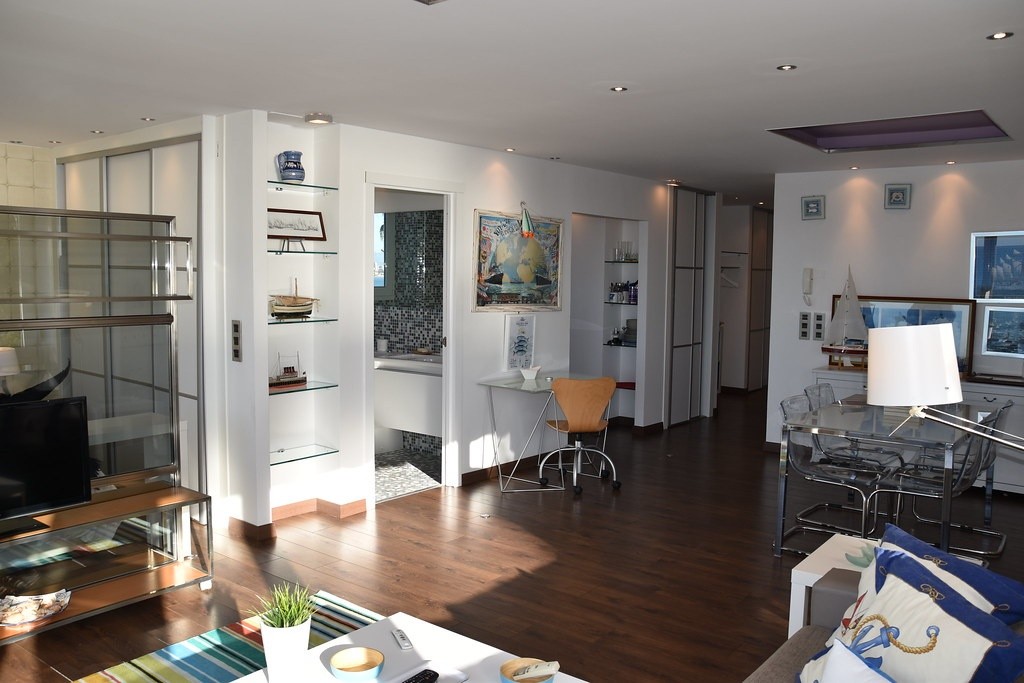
[[230, 612, 591, 683]]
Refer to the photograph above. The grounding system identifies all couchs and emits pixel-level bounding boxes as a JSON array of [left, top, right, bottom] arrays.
[[740, 533, 1024, 683]]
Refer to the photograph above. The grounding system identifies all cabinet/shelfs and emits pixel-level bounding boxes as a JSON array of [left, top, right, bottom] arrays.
[[267, 314, 338, 467], [603, 259, 640, 348], [810, 366, 1024, 496], [0, 485, 213, 647]]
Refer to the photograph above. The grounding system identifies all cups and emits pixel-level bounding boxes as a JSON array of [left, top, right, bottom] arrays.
[[622, 241, 632, 260], [613, 248, 621, 260], [618, 293, 623, 301], [377, 339, 388, 351], [609, 293, 617, 302]]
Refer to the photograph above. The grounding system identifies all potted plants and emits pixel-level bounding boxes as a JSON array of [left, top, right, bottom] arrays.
[[240, 578, 322, 683]]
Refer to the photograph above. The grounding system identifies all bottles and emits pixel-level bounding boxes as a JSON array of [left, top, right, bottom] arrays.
[[611, 280, 638, 303], [613, 327, 625, 344]]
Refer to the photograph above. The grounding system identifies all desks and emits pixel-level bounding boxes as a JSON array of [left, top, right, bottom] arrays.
[[774, 394, 997, 569], [478, 376, 595, 492]]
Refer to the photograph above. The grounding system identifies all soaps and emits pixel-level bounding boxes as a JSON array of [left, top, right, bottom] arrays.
[[416, 347, 428, 352]]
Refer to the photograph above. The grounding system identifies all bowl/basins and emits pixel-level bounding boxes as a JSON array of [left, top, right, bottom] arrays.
[[500, 658, 554, 683], [521, 366, 541, 380], [330, 647, 385, 682]]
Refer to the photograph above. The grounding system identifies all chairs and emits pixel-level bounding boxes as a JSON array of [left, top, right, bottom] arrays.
[[768, 383, 1014, 557], [539, 376, 621, 494]]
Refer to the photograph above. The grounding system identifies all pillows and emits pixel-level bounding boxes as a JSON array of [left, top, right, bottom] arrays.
[[795, 522, 1024, 683]]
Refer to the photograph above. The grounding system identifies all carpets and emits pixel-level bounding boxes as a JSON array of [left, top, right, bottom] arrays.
[[69, 590, 386, 683]]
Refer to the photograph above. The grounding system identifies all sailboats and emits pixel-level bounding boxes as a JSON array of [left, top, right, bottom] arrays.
[[820, 264, 869, 357]]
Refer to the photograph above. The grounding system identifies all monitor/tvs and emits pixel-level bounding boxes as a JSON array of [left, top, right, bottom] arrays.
[[0, 396, 91, 539]]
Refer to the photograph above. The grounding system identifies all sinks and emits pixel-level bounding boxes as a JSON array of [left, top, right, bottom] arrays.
[[387, 354, 442, 364]]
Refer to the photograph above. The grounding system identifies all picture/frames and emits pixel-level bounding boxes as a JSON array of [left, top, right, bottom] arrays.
[[884, 184, 911, 209], [801, 196, 826, 220], [471, 208, 565, 312], [267, 208, 327, 240], [504, 314, 537, 372], [969, 230, 1024, 303], [981, 305, 1024, 359], [829, 294, 976, 378]]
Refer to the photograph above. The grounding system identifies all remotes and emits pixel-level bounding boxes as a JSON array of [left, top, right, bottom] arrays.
[[511, 661, 560, 681], [402, 669, 439, 683], [391, 629, 412, 649]]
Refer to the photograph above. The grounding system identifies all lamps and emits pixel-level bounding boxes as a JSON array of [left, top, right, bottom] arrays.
[[304, 114, 333, 124], [865, 323, 1024, 451]]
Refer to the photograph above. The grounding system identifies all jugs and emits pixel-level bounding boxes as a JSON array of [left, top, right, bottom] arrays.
[[278, 151, 305, 183]]
